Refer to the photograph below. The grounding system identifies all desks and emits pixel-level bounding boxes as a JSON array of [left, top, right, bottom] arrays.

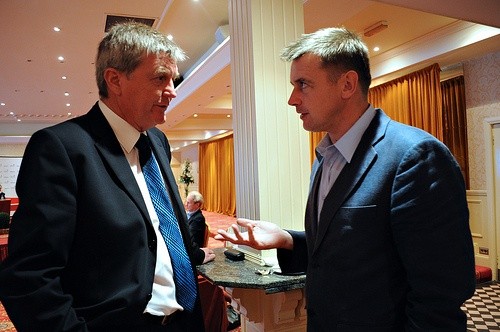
[[0, 199, 11, 218], [196, 246, 307, 294]]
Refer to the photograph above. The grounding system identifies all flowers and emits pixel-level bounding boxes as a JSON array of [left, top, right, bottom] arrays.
[[179, 158, 194, 199]]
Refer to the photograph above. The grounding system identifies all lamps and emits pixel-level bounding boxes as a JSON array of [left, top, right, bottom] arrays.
[[363, 21, 388, 37]]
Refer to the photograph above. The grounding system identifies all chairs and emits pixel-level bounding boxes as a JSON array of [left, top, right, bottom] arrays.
[[204, 224, 210, 248]]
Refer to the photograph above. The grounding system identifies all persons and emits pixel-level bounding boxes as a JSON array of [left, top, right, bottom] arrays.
[[185, 191, 205, 248], [0, 22, 216, 332], [214, 28, 476, 332]]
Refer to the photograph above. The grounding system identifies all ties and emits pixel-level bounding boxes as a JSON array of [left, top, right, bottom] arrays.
[[135, 133, 198, 312]]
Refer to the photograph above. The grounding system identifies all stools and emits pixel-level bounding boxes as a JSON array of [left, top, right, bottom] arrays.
[[474, 265, 492, 284]]
